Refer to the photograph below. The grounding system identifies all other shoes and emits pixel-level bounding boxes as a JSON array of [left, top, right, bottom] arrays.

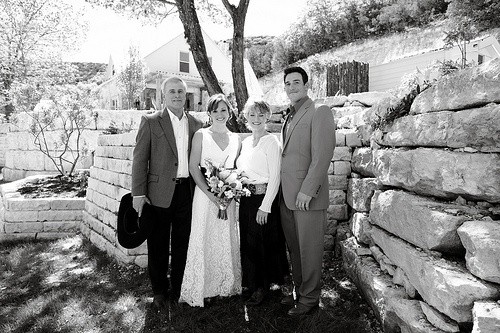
[[281, 283, 294, 296], [153, 295, 165, 315], [167, 299, 178, 317], [281, 294, 299, 304], [243, 288, 266, 307], [288, 297, 319, 316]]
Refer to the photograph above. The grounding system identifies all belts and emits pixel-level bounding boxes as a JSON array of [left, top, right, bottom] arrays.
[[176, 178, 189, 184], [242, 183, 267, 194]]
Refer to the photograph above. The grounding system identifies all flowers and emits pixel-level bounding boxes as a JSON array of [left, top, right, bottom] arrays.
[[204, 154, 256, 221]]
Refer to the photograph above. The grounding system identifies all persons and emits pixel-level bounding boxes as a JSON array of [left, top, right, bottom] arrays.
[[178, 95, 242, 307], [196, 101, 205, 112], [279, 67, 336, 316], [130, 76, 202, 303], [235, 97, 293, 303]]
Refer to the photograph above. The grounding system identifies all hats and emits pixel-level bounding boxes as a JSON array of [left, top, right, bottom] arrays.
[[117, 193, 153, 248]]
